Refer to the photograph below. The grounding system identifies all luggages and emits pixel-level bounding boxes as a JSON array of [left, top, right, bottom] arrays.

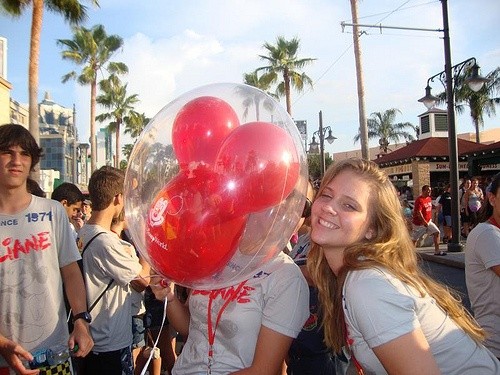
[[443, 236, 448, 244]]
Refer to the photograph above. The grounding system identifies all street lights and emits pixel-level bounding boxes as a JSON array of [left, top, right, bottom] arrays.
[[308, 125, 339, 180], [417, 57, 492, 253]]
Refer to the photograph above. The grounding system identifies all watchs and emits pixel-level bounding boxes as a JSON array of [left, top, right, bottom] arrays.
[[72, 312, 91, 325]]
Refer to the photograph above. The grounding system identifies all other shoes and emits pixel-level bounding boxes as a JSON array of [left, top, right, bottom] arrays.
[[434, 251, 447, 256]]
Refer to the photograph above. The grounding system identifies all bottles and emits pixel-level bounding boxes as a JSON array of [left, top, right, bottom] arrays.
[[21, 343, 78, 373]]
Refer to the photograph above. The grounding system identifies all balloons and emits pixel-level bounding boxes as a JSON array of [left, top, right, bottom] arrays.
[[123, 82, 308, 290]]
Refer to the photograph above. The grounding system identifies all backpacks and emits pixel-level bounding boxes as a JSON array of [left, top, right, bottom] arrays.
[[62, 232, 115, 334]]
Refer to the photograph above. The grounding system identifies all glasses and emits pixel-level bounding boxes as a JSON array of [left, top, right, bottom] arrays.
[[286, 188, 312, 218]]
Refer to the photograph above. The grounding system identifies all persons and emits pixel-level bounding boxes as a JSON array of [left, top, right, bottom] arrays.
[[69, 193, 92, 233], [465, 173, 500, 361], [395, 185, 414, 202], [26, 177, 46, 198], [51, 181, 84, 243], [402, 199, 412, 216], [150, 169, 316, 375], [431, 176, 485, 243], [77, 165, 188, 375], [482, 176, 493, 196], [412, 185, 447, 256], [283, 178, 336, 375], [0, 123, 95, 375], [307, 156, 500, 375]]
